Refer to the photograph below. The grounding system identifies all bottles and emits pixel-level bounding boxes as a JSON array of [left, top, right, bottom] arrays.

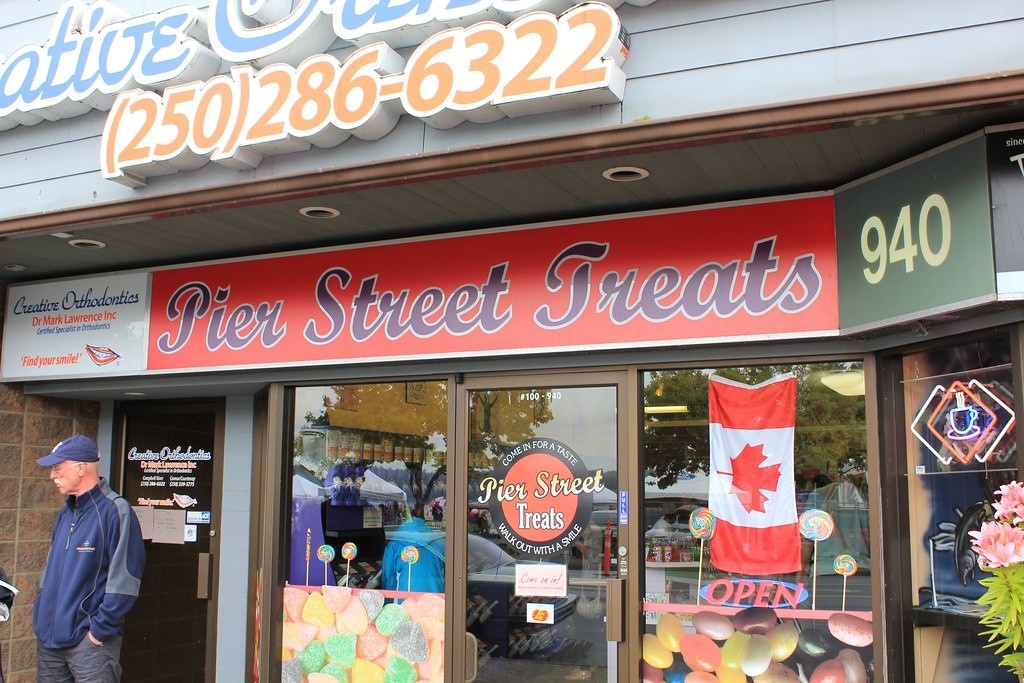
[[326, 436, 498, 506], [664, 543, 672, 562], [654, 540, 662, 563]]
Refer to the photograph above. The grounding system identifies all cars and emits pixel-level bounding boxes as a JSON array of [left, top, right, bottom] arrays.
[[328, 525, 579, 660]]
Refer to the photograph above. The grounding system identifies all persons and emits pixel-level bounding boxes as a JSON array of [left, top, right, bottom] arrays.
[[470, 510, 489, 534], [34, 434, 143, 683], [566, 519, 604, 681], [0, 567, 18, 683], [379, 503, 403, 544], [432, 501, 444, 522], [797, 454, 871, 641], [382, 508, 446, 605]]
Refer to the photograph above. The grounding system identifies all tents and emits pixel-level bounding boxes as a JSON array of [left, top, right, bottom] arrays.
[[587, 462, 708, 504], [292, 468, 409, 525]]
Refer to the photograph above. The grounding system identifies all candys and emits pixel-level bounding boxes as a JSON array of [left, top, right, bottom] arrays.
[[401, 545, 420, 564], [688, 507, 717, 541], [341, 542, 357, 560], [832, 555, 857, 576], [642, 605, 874, 683], [281, 585, 445, 683], [798, 508, 835, 542], [305, 528, 311, 561], [317, 544, 335, 562]]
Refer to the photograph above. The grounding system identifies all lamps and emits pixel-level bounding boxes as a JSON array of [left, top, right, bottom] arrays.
[[641, 404, 692, 414]]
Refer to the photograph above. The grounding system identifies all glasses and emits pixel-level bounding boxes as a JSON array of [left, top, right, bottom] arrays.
[[52, 461, 88, 475]]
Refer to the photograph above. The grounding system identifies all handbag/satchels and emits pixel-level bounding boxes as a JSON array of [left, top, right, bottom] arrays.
[[577, 593, 602, 619]]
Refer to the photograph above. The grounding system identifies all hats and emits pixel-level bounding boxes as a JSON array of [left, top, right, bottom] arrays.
[[35, 434, 99, 466]]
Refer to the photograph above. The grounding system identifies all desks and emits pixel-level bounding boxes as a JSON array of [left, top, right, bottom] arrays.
[[466, 572, 609, 591]]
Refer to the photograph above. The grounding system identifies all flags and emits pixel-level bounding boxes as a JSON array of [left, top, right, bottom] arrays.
[[708, 373, 802, 576]]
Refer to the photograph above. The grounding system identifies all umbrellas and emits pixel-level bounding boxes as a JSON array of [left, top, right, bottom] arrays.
[[427, 497, 446, 515]]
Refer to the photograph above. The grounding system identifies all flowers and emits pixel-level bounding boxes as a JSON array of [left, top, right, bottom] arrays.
[[967, 480, 1024, 683]]
